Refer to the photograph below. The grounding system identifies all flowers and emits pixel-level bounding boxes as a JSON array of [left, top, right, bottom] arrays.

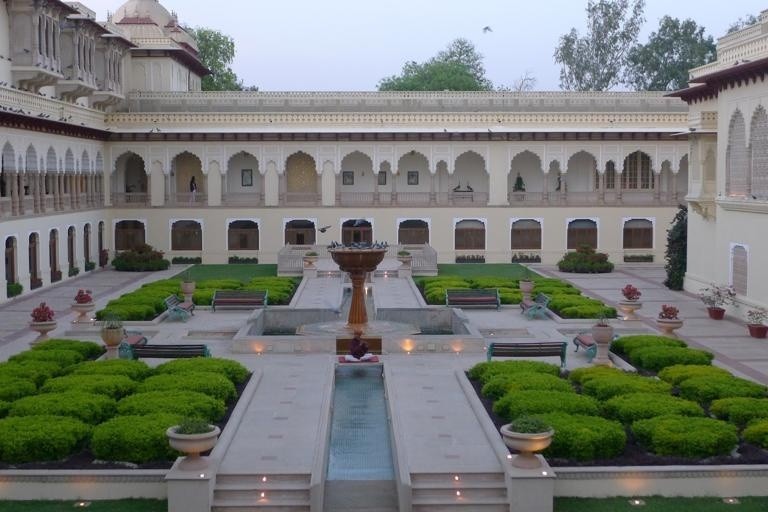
[[698, 281, 740, 309], [658, 304, 680, 319], [746, 307, 767, 324], [30, 302, 53, 321], [73, 288, 92, 303], [621, 283, 642, 301]]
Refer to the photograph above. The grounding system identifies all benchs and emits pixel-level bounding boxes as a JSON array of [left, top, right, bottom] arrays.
[[127, 343, 211, 361], [487, 341, 567, 369], [445, 287, 501, 312], [519, 292, 549, 320], [573, 332, 598, 364], [120, 328, 147, 345], [210, 288, 268, 313], [163, 293, 196, 321]]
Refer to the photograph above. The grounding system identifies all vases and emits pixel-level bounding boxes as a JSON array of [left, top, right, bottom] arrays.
[[656, 318, 682, 338], [706, 306, 725, 320], [70, 303, 95, 324], [27, 320, 58, 343], [617, 300, 642, 321], [747, 323, 767, 337]]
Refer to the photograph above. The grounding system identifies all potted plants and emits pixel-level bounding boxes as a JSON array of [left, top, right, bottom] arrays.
[[395, 250, 412, 270], [519, 265, 535, 300], [100, 312, 128, 346], [165, 412, 221, 470], [591, 310, 614, 344], [177, 267, 197, 296], [301, 251, 320, 270], [500, 415, 557, 468]]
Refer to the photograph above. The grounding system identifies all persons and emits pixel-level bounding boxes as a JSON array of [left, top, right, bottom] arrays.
[[512, 173, 525, 192], [189, 176, 197, 201], [555, 172, 561, 191]]
